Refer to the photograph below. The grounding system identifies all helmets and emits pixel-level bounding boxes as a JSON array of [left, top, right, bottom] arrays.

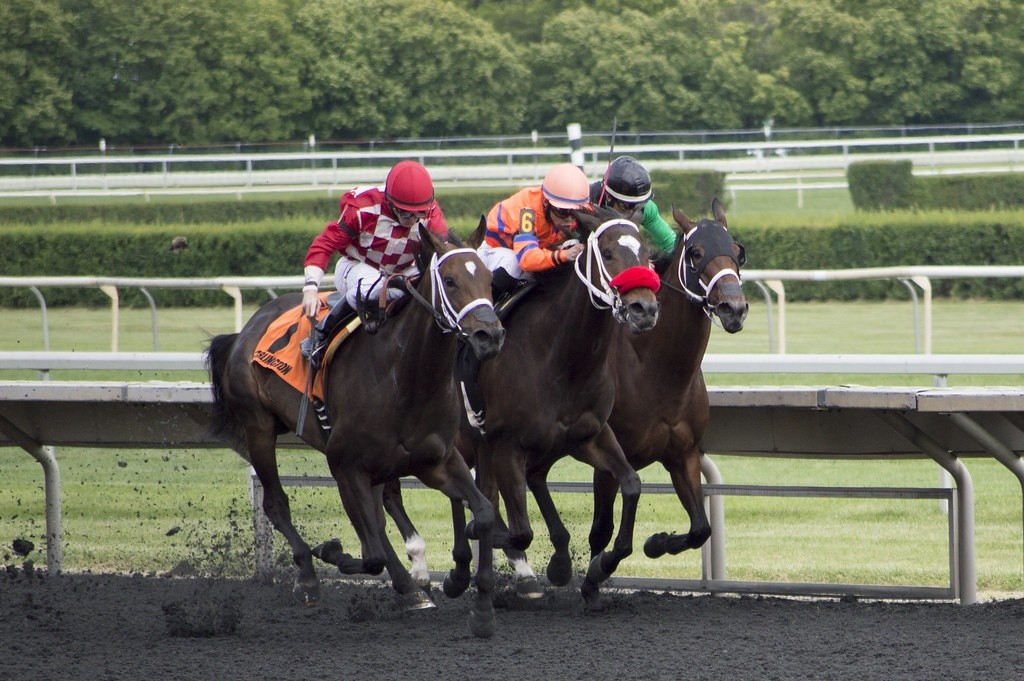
[[601, 155, 655, 203], [385, 159, 436, 211], [542, 163, 591, 210]]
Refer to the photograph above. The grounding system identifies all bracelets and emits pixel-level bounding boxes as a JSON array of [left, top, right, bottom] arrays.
[[304, 280, 319, 288]]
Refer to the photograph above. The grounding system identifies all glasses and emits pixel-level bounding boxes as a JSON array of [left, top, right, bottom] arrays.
[[613, 197, 647, 211], [550, 205, 578, 220], [392, 207, 429, 219]]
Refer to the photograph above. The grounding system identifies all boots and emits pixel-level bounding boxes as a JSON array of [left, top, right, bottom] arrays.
[[300, 293, 358, 369]]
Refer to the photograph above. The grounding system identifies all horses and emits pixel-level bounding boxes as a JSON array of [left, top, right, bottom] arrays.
[[201, 196, 749, 638]]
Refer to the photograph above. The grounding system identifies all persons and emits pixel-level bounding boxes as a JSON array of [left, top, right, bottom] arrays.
[[589, 156, 677, 255], [476, 164, 593, 305], [299, 161, 451, 370]]
